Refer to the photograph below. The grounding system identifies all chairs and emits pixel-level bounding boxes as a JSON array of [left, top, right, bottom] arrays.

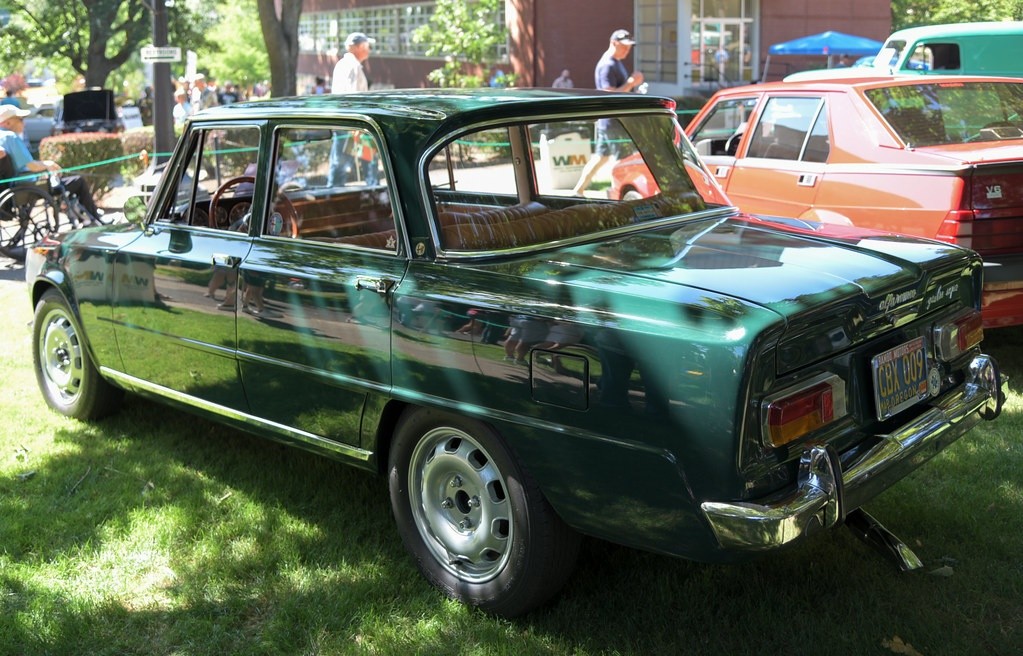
[[890, 106, 932, 146], [759, 119, 812, 164]]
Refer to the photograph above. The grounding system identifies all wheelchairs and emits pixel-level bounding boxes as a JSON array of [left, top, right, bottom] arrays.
[[0, 151, 116, 264]]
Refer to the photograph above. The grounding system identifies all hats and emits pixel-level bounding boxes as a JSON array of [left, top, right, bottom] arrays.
[[345, 32, 376, 46], [0, 104, 31, 123], [610, 29, 636, 46]]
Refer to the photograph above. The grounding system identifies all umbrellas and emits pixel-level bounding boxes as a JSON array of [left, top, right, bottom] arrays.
[[769, 30, 883, 68]]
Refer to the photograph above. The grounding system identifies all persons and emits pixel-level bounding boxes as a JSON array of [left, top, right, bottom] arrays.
[[571, 29, 647, 198], [206, 242, 670, 411], [326, 32, 380, 189], [553, 69, 574, 88], [836, 54, 849, 70], [0, 75, 330, 224]]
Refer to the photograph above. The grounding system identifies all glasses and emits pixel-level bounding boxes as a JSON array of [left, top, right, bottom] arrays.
[[9, 117, 23, 122]]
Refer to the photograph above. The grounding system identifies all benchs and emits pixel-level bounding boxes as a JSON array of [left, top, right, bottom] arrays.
[[309, 190, 704, 257]]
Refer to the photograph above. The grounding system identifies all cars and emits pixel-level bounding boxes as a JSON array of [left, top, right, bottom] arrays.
[[31, 86, 1011, 617], [874, 18, 1023, 80], [19, 86, 145, 145], [604, 71, 1023, 332]]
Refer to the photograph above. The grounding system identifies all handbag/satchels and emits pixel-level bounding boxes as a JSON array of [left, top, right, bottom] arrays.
[[343, 130, 376, 163]]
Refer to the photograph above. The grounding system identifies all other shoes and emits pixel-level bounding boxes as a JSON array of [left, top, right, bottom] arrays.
[[98, 218, 114, 225]]
